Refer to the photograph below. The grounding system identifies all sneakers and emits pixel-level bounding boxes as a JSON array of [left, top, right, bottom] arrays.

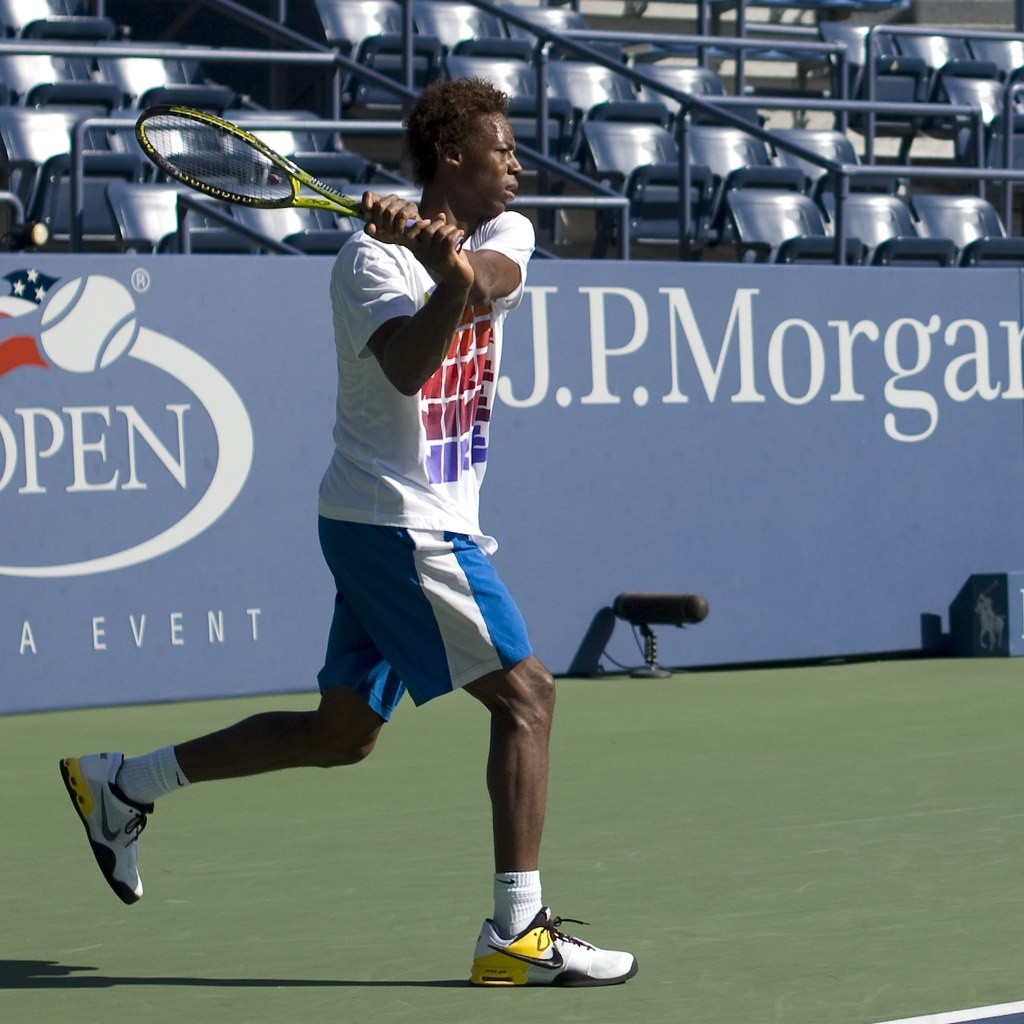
[[470, 906, 640, 988], [58, 753, 155, 905]]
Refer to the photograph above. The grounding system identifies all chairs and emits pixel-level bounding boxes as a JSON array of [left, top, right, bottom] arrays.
[[0, 0, 1023, 271]]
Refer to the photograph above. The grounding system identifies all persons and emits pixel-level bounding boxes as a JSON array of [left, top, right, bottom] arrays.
[[60, 77, 638, 988]]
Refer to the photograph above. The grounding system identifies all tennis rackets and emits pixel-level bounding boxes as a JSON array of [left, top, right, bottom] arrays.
[[135, 104, 465, 256]]
